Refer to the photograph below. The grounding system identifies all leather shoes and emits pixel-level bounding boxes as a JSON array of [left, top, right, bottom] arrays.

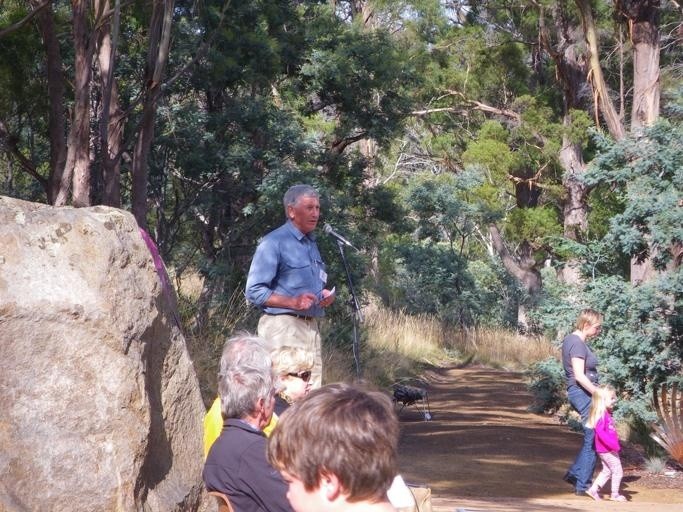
[[563, 471, 577, 487]]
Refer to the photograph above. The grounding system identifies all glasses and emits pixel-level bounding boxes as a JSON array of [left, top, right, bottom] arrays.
[[288, 370, 312, 379]]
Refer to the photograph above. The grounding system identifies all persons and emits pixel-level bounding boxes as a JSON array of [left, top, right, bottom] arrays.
[[585, 384, 627, 501], [203, 336, 277, 463], [202, 365, 292, 512], [561, 309, 602, 496], [264, 382, 400, 512], [270, 345, 314, 418], [245, 185, 335, 404]]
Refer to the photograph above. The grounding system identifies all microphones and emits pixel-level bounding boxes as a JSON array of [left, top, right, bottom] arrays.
[[321, 223, 352, 247]]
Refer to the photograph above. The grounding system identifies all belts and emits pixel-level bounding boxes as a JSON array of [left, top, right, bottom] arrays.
[[285, 312, 313, 320]]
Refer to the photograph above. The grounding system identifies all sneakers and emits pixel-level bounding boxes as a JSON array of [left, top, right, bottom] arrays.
[[585, 487, 601, 501], [610, 492, 628, 502]]
